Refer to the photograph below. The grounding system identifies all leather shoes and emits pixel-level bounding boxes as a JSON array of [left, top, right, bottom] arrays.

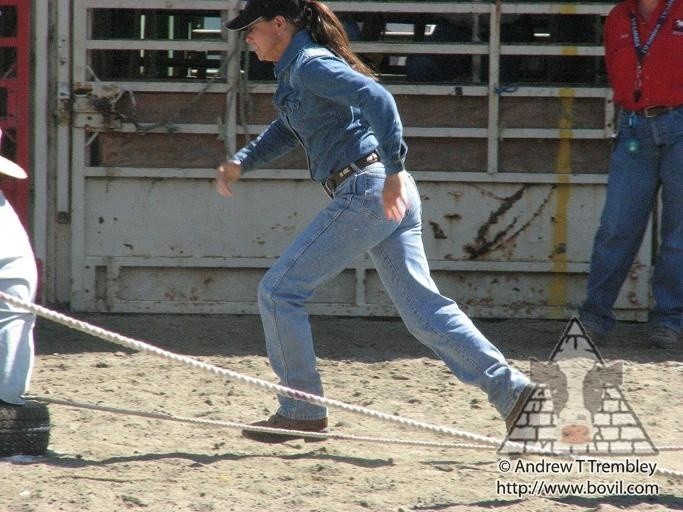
[[241, 416, 326, 443], [649, 326, 678, 349], [507, 384, 545, 460]]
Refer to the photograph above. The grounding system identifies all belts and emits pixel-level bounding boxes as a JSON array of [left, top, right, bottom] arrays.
[[629, 106, 678, 116], [323, 152, 381, 198]]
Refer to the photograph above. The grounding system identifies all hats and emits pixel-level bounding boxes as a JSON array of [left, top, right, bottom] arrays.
[[0, 130, 28, 179], [222, 0, 304, 32]]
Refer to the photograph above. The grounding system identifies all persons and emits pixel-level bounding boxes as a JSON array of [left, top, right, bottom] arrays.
[[211, 0, 545, 462], [1, 151, 39, 409], [579, 1, 683, 348], [327, 1, 617, 89]]
